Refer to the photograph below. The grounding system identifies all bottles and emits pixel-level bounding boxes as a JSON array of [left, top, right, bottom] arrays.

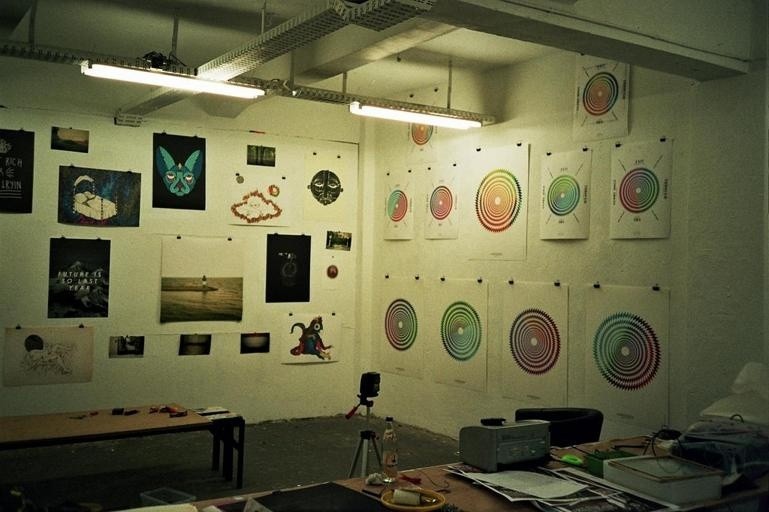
[[381, 415, 398, 483]]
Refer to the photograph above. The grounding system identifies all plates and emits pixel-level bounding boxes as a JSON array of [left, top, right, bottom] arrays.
[[380, 486, 446, 512]]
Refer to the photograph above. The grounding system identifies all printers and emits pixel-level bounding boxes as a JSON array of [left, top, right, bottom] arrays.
[[459, 420, 550, 472]]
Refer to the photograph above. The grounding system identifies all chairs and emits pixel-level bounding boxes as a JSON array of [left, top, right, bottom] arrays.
[[514, 408, 605, 444]]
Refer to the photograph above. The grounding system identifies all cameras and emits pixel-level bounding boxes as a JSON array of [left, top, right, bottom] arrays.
[[358, 369, 382, 408]]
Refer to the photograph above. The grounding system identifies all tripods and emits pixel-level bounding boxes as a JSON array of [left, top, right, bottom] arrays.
[[348, 406, 383, 479]]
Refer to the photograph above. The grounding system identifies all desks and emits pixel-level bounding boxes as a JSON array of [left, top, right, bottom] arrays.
[[0, 403, 246, 490], [113, 435, 769, 511]]
